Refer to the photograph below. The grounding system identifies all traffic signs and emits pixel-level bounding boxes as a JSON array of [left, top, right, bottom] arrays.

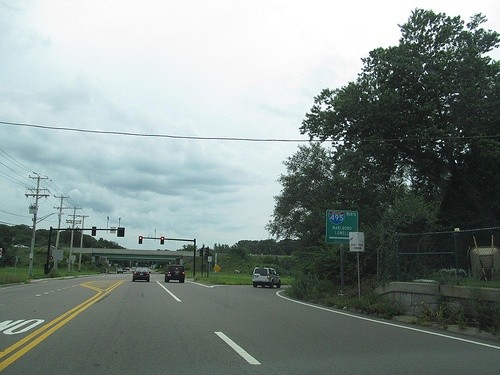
[[325, 209, 359, 245]]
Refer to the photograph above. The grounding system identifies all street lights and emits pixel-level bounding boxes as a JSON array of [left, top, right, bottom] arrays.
[[29, 212, 64, 278]]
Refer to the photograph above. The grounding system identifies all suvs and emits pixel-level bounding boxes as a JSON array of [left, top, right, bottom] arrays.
[[165, 266, 185, 283], [252, 267, 282, 289]]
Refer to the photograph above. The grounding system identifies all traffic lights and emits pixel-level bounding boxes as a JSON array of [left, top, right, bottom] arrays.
[[199, 248, 203, 257], [205, 248, 209, 257], [139, 236, 143, 244], [160, 237, 164, 245]]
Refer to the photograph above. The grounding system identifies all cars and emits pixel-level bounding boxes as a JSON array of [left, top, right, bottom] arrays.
[[117, 267, 136, 274], [132, 267, 151, 282]]
